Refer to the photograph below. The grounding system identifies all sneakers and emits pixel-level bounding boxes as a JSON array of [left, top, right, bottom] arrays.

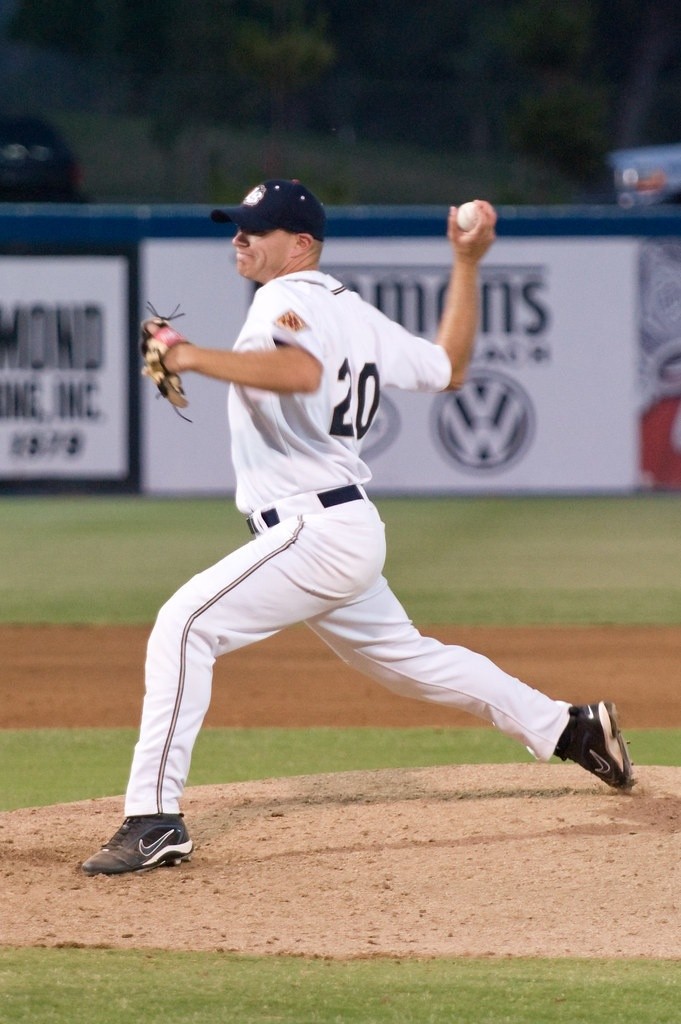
[[553, 701, 635, 790], [83, 812, 194, 876]]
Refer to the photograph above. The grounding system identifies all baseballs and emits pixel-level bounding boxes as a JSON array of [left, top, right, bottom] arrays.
[[455, 201, 479, 233]]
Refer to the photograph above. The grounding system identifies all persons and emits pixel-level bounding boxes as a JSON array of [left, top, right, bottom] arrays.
[[80, 181, 637, 875]]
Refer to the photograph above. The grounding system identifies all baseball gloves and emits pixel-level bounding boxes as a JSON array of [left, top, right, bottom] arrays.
[[138, 315, 191, 408]]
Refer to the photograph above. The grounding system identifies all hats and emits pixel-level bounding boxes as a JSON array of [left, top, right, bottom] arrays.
[[212, 179, 326, 242]]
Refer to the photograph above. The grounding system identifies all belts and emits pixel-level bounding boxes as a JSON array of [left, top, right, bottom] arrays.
[[246, 483, 364, 535]]
